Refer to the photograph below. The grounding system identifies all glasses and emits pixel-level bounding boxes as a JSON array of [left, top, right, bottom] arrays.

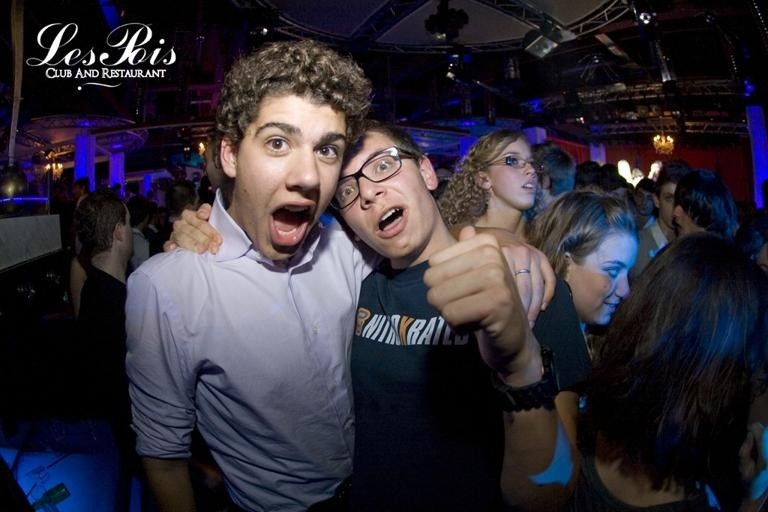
[[330, 145, 419, 210], [481, 156, 546, 173]]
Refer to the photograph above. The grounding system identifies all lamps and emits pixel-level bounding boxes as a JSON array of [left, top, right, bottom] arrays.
[[424, 0, 657, 104]]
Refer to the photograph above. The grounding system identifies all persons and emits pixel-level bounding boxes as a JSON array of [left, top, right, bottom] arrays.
[[1, 38, 767, 510]]
